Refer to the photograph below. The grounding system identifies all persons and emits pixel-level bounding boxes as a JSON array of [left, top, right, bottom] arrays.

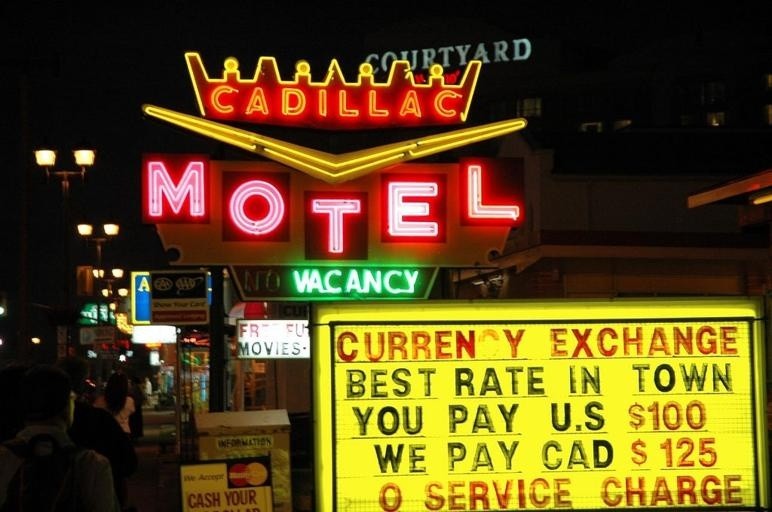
[[0, 361, 30, 442], [0, 361, 121, 512], [93, 371, 137, 434], [55, 362, 137, 511], [126, 378, 145, 445]]
